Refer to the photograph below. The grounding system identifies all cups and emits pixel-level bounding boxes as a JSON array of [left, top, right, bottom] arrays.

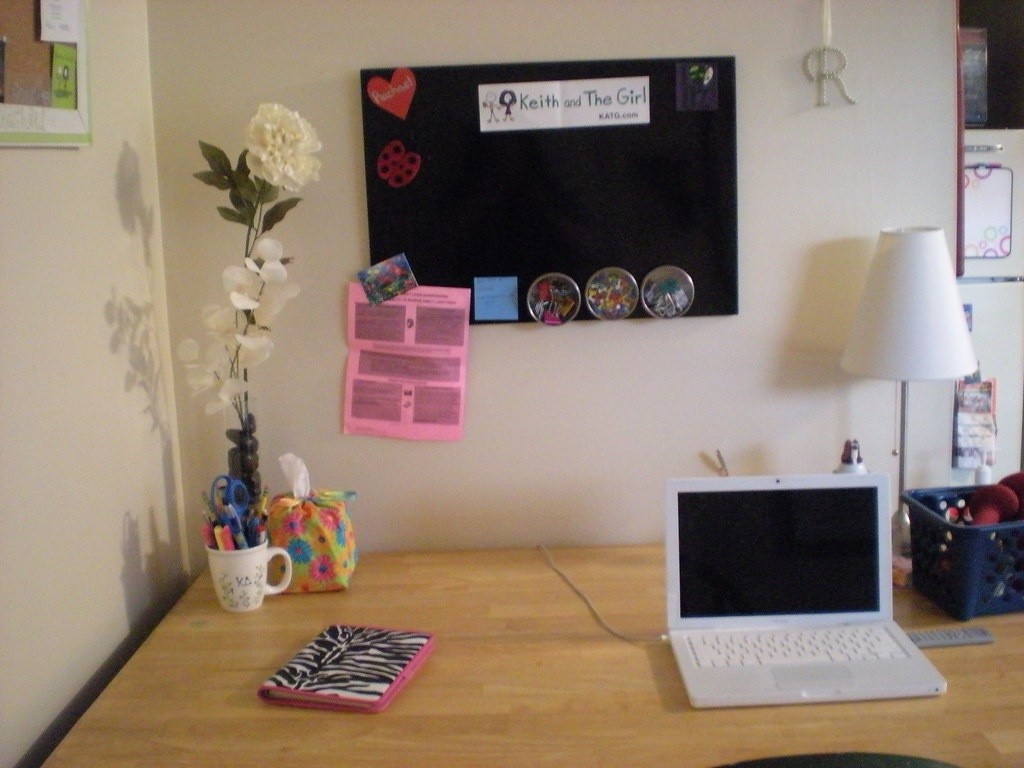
[[205, 538, 293, 613]]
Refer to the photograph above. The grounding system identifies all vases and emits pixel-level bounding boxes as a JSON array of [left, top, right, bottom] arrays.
[[226, 413, 262, 508]]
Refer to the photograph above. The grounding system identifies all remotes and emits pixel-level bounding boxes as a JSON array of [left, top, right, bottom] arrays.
[[905, 628, 993, 648]]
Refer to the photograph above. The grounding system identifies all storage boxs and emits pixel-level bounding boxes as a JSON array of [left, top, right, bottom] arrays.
[[956, 27, 990, 127], [900, 483, 1024, 621]]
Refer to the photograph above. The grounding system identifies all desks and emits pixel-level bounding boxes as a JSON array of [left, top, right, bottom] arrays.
[[45, 544, 1024, 767]]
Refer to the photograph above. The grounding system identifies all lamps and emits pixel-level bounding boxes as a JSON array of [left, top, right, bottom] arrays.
[[836, 225, 978, 535]]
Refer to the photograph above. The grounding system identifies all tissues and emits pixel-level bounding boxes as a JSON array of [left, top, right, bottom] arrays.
[[263, 453, 361, 594]]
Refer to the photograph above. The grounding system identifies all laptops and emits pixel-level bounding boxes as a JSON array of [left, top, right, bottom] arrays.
[[665, 472, 948, 708]]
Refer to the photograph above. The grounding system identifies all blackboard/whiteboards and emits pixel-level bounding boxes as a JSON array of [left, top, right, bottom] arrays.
[[356, 55, 741, 328]]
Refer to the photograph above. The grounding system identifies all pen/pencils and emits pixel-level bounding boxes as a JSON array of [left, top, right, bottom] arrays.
[[198, 485, 271, 552]]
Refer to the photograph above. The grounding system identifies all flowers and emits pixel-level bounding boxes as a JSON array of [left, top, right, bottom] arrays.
[[176, 99, 342, 418]]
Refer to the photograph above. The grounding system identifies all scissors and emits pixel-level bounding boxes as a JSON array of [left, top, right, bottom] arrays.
[[208, 473, 252, 523]]
